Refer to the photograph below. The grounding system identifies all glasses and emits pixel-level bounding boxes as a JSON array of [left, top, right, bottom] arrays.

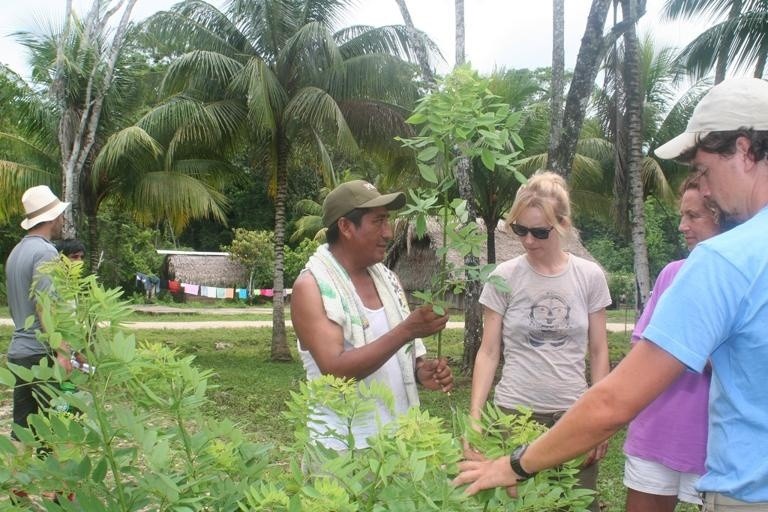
[[509, 219, 561, 240]]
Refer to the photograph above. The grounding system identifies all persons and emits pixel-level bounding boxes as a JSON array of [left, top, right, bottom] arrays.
[[462, 167, 613, 512], [289, 178, 455, 512], [55, 236, 112, 353], [447, 75, 766, 512], [622, 175, 735, 512], [4, 182, 89, 511]]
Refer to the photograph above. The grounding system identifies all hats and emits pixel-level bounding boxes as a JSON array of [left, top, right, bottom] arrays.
[[321, 179, 407, 228], [19, 185, 71, 231], [652, 76, 768, 163]]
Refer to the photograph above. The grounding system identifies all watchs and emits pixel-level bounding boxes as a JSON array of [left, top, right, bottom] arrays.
[[509, 443, 539, 482]]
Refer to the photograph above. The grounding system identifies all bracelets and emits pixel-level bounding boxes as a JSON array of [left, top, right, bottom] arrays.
[[415, 366, 425, 386], [71, 350, 79, 358]]
